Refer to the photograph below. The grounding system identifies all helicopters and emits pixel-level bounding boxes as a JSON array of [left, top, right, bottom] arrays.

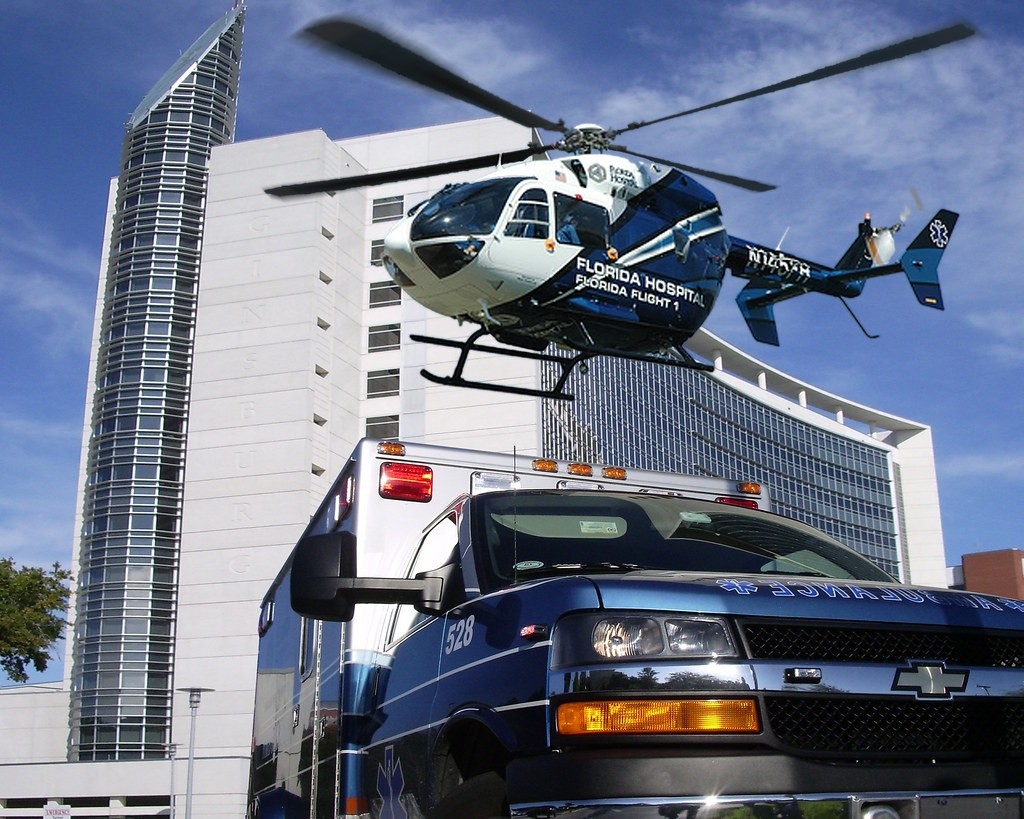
[[262, 14, 976, 401]]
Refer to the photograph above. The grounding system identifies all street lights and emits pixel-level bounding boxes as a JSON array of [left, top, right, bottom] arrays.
[[176, 687, 215, 819]]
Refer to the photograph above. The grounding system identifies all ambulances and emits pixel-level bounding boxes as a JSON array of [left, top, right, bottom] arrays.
[[245, 439, 1024, 819]]
